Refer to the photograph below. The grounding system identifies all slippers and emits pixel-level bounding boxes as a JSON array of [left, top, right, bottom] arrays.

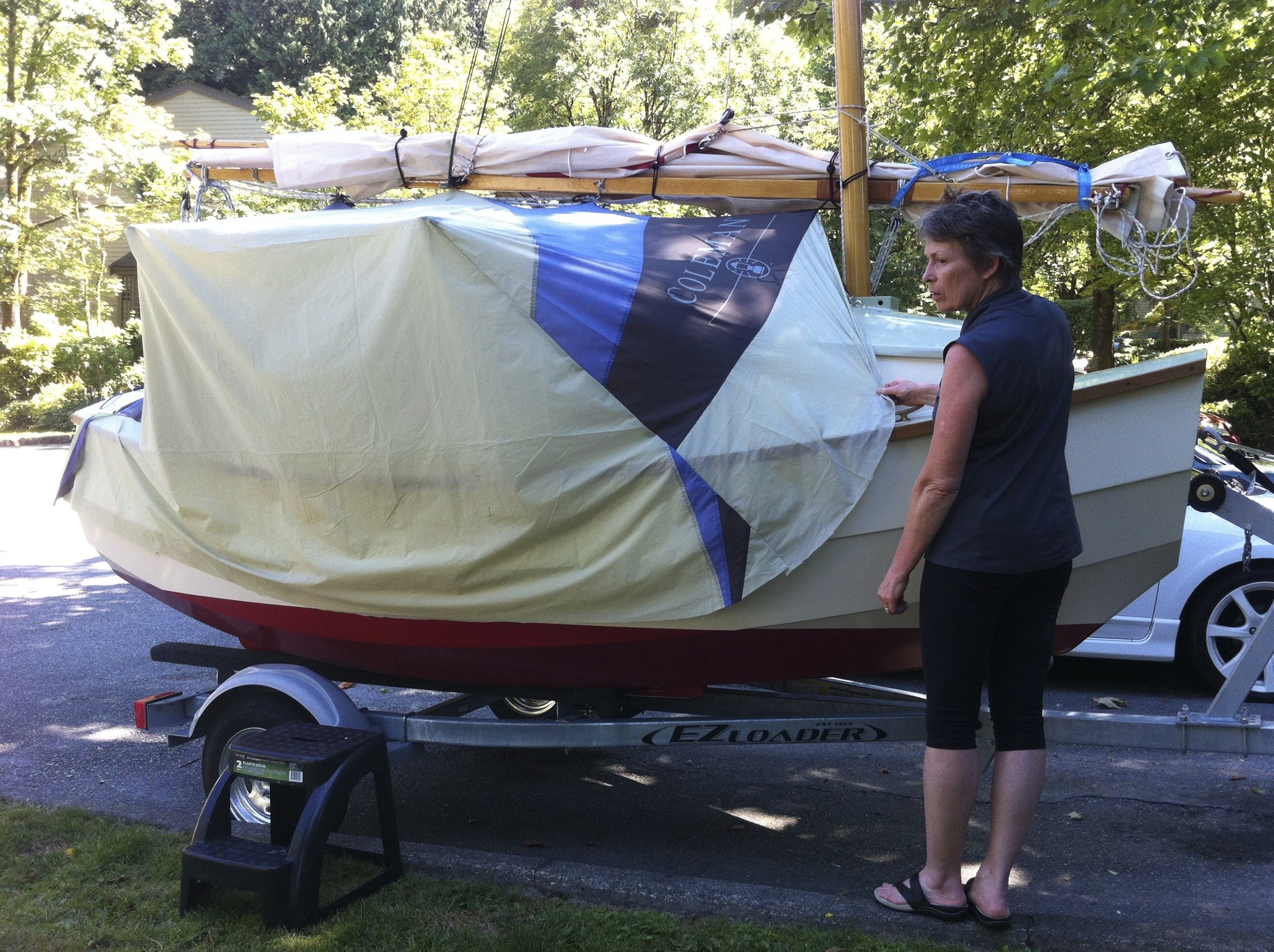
[[963, 876, 1013, 930], [874, 874, 970, 922]]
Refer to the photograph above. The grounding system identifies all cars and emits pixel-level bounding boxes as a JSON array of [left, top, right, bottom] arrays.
[[1071, 435, 1274, 702]]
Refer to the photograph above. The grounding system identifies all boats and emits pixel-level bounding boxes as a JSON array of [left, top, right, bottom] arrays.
[[67, 346, 1205, 674]]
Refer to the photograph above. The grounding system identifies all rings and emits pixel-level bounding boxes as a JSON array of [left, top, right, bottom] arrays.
[[884, 607, 889, 611]]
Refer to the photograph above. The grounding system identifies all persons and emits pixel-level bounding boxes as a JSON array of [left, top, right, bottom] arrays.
[[876, 190, 1081, 927]]
